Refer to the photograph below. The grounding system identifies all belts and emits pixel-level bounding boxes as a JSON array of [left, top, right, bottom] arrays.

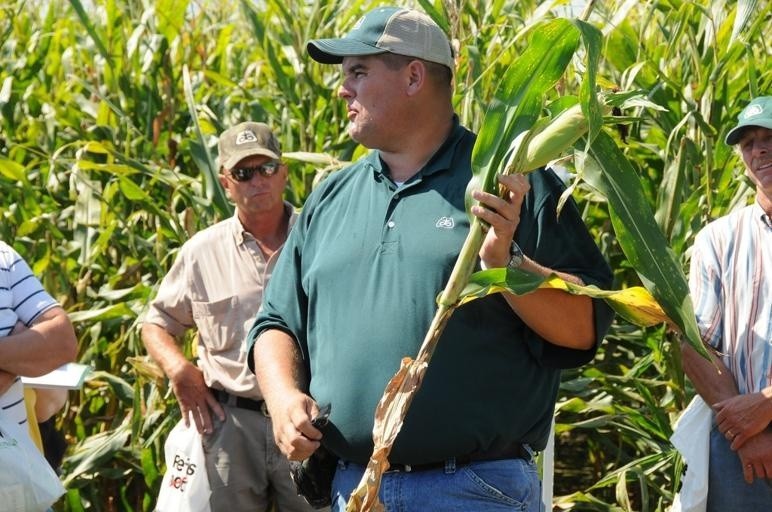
[[342, 442, 531, 472], [206, 387, 272, 417]]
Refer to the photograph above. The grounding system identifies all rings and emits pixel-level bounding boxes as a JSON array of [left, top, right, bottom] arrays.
[[728, 430, 735, 438]]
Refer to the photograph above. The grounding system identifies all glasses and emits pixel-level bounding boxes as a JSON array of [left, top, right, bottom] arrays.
[[224, 160, 286, 184]]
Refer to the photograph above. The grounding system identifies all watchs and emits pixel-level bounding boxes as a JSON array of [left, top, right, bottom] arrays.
[[480, 239, 525, 279]]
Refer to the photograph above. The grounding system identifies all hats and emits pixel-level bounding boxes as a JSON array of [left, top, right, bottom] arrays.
[[307, 6, 457, 73], [216, 122, 281, 171], [724, 95, 772, 146]]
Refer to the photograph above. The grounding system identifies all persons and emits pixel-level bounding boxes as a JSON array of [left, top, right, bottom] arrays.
[[139, 122, 335, 512], [681, 96, 772, 512], [245, 6, 615, 512], [0, 240, 79, 512]]
